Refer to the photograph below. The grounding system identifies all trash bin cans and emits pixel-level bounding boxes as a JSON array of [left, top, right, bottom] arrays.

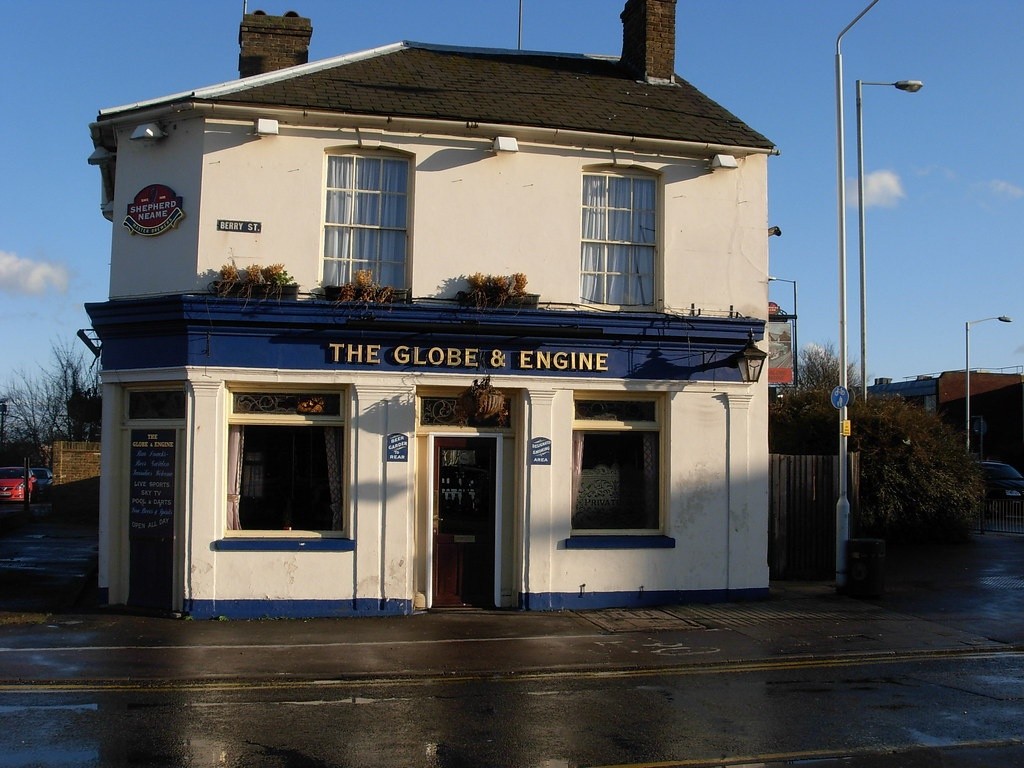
[[845, 537, 887, 602]]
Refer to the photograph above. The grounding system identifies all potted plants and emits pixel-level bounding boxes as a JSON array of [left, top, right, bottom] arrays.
[[454, 274, 541, 310], [322, 268, 413, 303], [208, 263, 301, 301]]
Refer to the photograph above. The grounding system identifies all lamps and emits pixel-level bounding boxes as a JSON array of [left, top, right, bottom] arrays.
[[128, 123, 163, 141], [711, 153, 739, 170], [253, 119, 281, 137], [491, 136, 521, 153]]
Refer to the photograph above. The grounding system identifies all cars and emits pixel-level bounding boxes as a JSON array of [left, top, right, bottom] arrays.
[[0, 467, 37, 504], [30, 468, 54, 494], [969, 461, 1024, 518]]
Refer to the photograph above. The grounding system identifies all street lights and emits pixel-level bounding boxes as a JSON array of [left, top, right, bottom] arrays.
[[769, 275, 798, 389], [965, 315, 1013, 452], [856, 79, 923, 536]]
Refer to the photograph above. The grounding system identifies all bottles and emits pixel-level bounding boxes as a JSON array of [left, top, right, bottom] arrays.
[[440, 478, 446, 492], [458, 478, 463, 505], [444, 478, 450, 500], [469, 480, 476, 500]]
